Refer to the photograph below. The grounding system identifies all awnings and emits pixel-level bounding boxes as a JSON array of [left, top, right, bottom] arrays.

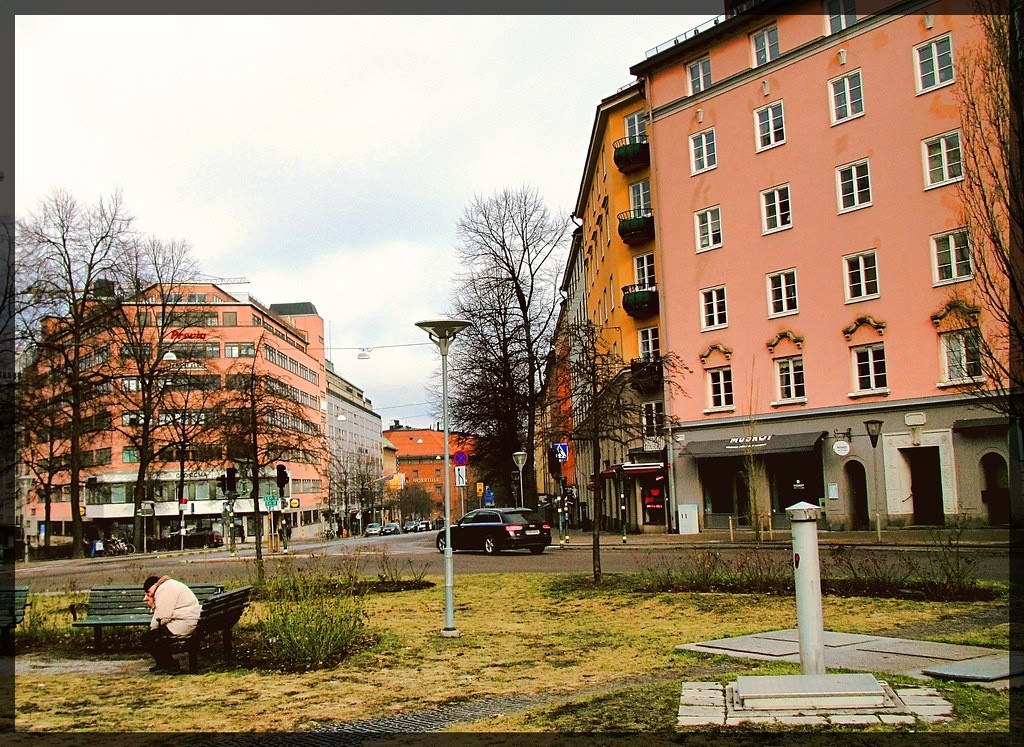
[[622, 462, 664, 474], [677, 432, 822, 458], [602, 466, 621, 479]]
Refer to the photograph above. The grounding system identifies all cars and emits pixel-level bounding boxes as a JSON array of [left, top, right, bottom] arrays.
[[382, 523, 400, 535], [419, 521, 433, 531], [175, 530, 225, 548], [402, 521, 419, 534], [365, 523, 383, 536]]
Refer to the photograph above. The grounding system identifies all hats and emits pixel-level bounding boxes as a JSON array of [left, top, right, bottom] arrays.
[[144, 576, 160, 593]]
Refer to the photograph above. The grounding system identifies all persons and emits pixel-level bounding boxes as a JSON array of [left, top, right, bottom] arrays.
[[278, 520, 291, 549], [426, 521, 430, 531], [85, 522, 100, 557], [104, 530, 112, 544], [141, 575, 200, 675], [240, 525, 245, 543]]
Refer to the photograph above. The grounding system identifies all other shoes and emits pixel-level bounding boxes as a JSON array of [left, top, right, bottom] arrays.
[[149, 664, 163, 672], [169, 664, 180, 674]]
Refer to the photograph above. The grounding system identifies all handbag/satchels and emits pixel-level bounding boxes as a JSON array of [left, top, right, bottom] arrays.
[[96, 540, 104, 551]]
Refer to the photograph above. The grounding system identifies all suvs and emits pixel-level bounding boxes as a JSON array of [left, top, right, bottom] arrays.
[[435, 507, 552, 556]]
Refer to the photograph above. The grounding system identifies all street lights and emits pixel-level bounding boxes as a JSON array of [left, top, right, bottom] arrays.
[[863, 416, 886, 545], [512, 451, 527, 508], [413, 318, 474, 639]]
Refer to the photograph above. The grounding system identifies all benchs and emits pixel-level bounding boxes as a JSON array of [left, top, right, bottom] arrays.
[[69, 583, 224, 656], [152, 585, 254, 674], [0, 585, 33, 629]]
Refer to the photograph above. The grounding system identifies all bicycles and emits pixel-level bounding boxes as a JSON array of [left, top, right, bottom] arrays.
[[104, 538, 136, 556]]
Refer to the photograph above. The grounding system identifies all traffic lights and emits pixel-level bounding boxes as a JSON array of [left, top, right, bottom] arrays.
[[226, 468, 243, 492], [216, 474, 227, 494], [276, 464, 289, 489], [281, 500, 290, 512]]
